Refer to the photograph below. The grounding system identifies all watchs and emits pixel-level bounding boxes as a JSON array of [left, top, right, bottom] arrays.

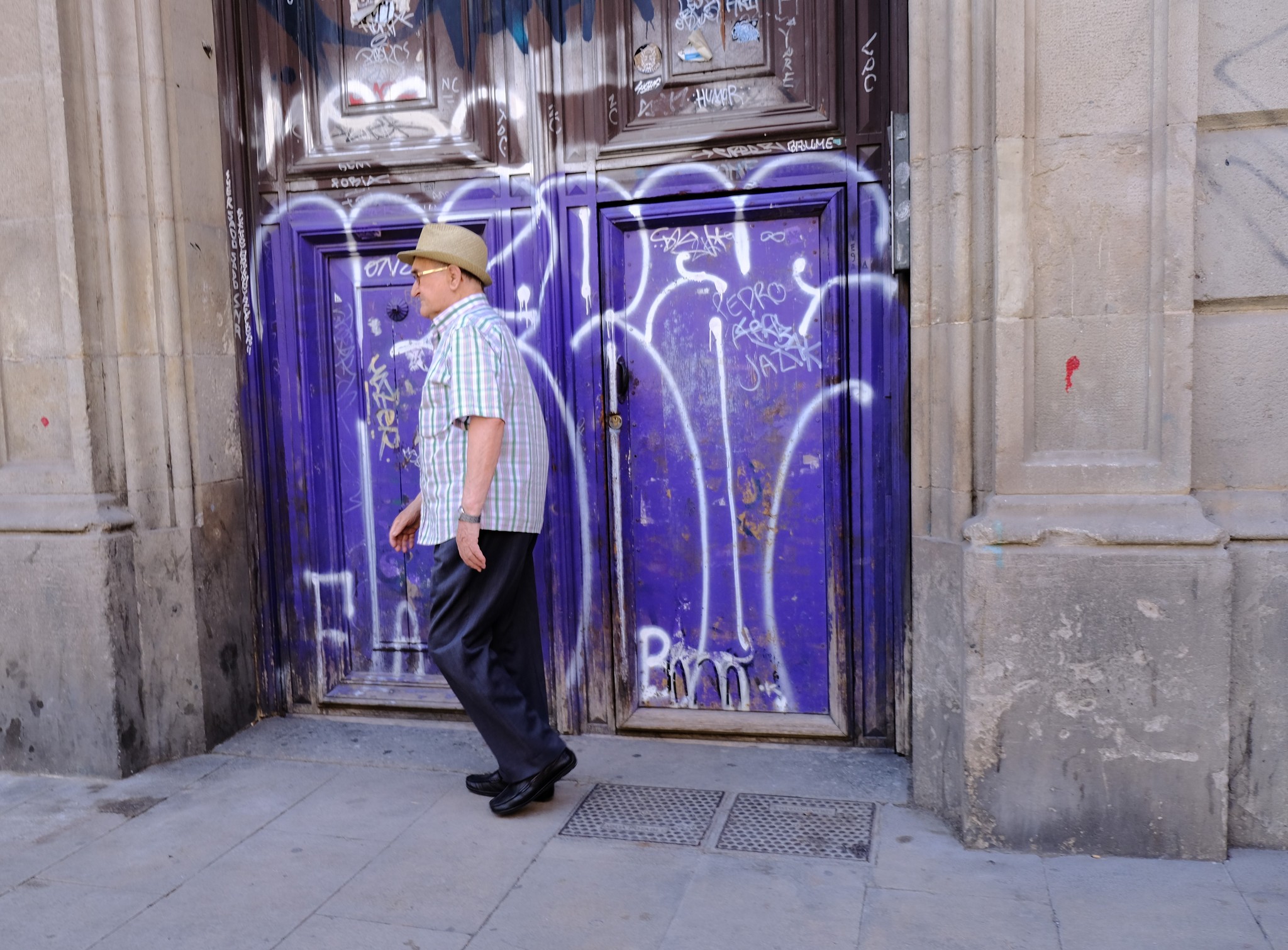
[[454, 507, 483, 525]]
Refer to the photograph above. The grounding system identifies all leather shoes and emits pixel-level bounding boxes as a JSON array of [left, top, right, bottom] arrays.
[[465, 767, 555, 802], [489, 746, 577, 815]]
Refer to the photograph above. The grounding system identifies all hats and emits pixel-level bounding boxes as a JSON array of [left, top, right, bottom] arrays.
[[396, 223, 493, 287]]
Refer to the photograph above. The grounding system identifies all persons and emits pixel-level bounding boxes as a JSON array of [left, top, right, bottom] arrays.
[[388, 219, 578, 816]]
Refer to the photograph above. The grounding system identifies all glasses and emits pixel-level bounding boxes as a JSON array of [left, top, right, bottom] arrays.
[[411, 265, 448, 285]]
